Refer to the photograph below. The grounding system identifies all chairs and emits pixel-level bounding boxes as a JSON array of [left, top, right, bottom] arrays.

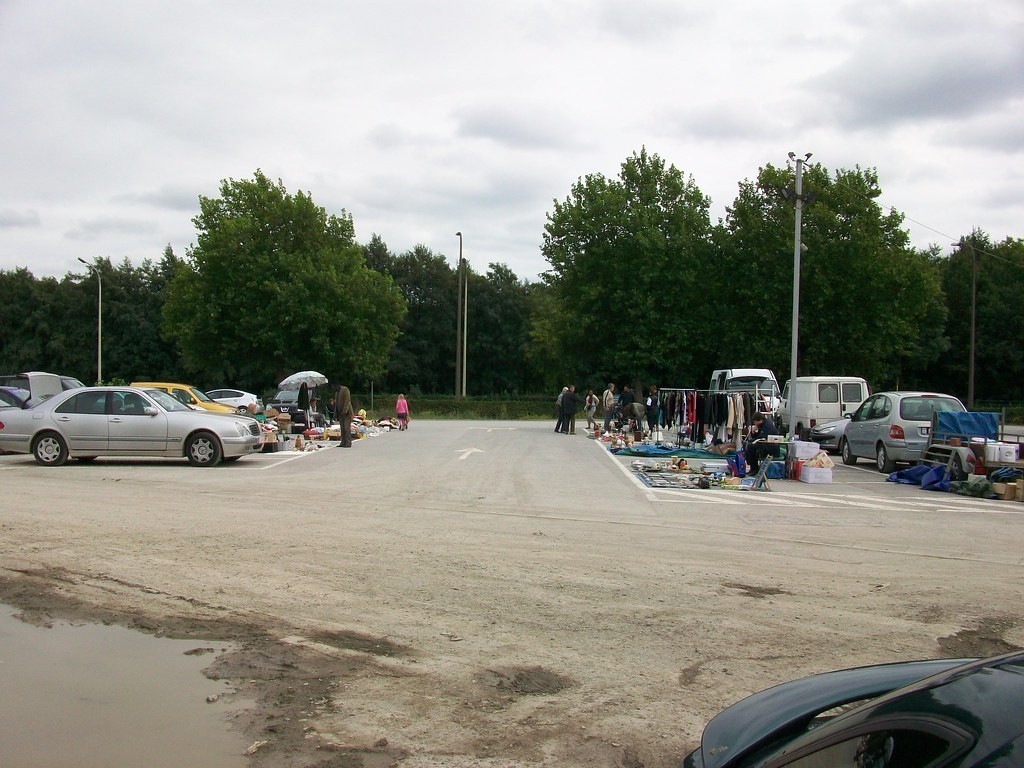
[[112, 400, 124, 415], [124, 395, 137, 415], [914, 405, 931, 419]]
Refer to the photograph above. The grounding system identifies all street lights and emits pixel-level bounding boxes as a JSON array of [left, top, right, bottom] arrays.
[[779, 186, 817, 440], [455, 231, 462, 396], [78, 255, 101, 386], [461, 257, 468, 397]]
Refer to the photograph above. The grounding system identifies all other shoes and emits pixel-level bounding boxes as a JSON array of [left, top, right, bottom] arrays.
[[746, 465, 760, 476], [585, 426, 590, 429], [555, 430, 561, 433], [570, 432, 576, 435], [337, 445, 351, 447]]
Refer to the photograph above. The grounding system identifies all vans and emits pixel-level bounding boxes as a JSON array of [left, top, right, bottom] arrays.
[[774, 377, 871, 443], [708, 366, 782, 428], [0, 372, 88, 392]]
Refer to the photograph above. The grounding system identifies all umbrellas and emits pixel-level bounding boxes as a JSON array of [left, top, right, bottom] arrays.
[[278, 371, 328, 390]]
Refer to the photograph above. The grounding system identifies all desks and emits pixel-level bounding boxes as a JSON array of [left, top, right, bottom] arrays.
[[755, 440, 808, 468]]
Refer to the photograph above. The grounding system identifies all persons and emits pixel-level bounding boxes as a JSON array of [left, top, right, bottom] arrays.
[[309, 398, 330, 427], [396, 394, 409, 431], [603, 383, 615, 430], [554, 387, 569, 433], [744, 412, 780, 476], [585, 389, 600, 429], [618, 384, 634, 417], [619, 413, 635, 427], [327, 382, 353, 447], [325, 399, 336, 424], [560, 385, 582, 435], [625, 402, 646, 430], [646, 385, 659, 436]]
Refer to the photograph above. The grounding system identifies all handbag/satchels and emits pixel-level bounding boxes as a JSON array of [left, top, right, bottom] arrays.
[[406, 415, 410, 424]]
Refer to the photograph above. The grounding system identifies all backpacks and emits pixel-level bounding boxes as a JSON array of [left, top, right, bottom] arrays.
[[727, 448, 746, 479]]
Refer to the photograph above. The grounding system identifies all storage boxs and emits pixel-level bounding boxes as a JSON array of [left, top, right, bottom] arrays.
[[993, 479, 1024, 502], [328, 432, 341, 440], [263, 409, 291, 427], [259, 438, 295, 453], [787, 442, 834, 484]]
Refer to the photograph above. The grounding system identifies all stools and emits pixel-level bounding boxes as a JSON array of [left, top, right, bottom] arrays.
[[756, 454, 785, 478], [307, 420, 318, 427]]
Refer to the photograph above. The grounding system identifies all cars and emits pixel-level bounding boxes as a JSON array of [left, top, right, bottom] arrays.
[[841, 391, 968, 475], [0, 385, 265, 467], [125, 382, 241, 414], [808, 400, 947, 455], [0, 386, 45, 412], [265, 388, 327, 429], [204, 389, 264, 417]]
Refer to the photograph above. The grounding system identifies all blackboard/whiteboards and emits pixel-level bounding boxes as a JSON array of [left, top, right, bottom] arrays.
[[755, 454, 773, 486]]
